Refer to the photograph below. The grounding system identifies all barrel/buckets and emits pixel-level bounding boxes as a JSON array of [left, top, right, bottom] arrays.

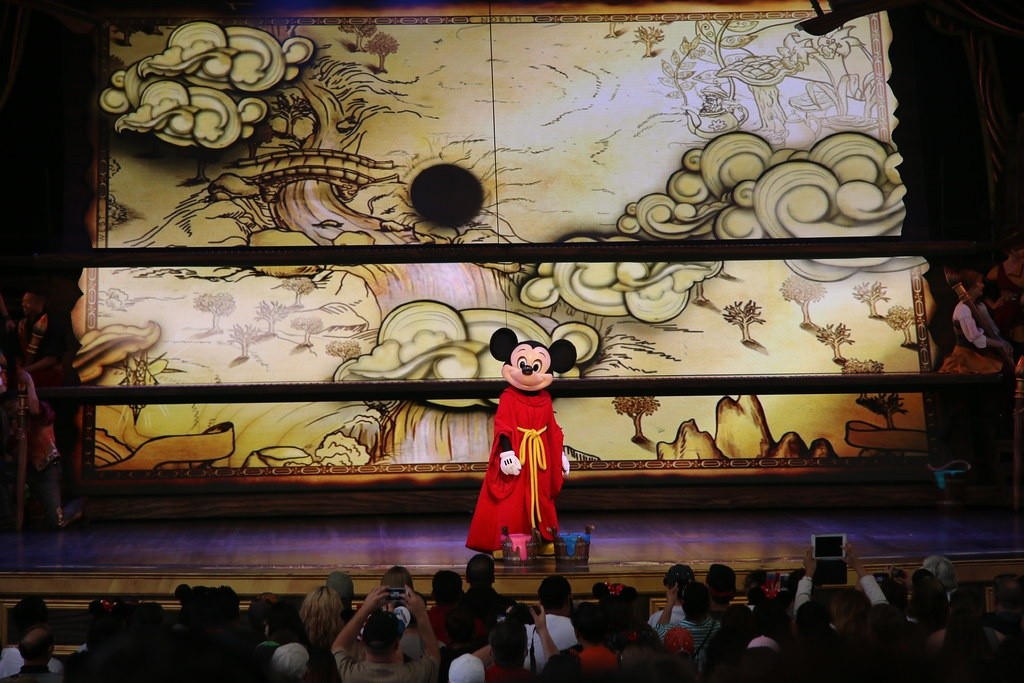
[[551, 524, 596, 563], [500, 524, 543, 565]]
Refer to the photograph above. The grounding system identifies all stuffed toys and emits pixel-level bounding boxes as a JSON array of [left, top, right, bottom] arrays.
[[466, 328, 577, 561]]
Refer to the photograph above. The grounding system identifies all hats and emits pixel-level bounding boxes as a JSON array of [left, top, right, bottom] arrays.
[[448, 653, 485, 683], [270, 643, 309, 680], [666, 627, 693, 655], [747, 635, 778, 653], [362, 611, 406, 650]]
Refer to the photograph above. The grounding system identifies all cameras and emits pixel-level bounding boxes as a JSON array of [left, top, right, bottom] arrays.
[[512, 604, 541, 624], [383, 587, 407, 602], [887, 566, 903, 578], [676, 589, 685, 598]]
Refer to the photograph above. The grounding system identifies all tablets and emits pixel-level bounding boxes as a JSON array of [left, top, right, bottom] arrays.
[[811, 533, 848, 560]]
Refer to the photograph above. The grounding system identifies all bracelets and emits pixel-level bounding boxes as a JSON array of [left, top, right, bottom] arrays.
[[5, 314, 11, 321]]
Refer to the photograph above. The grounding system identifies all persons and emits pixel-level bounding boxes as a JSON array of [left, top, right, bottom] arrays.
[[2, 539, 1024, 683], [0, 291, 81, 533], [925, 224, 1023, 376]]
